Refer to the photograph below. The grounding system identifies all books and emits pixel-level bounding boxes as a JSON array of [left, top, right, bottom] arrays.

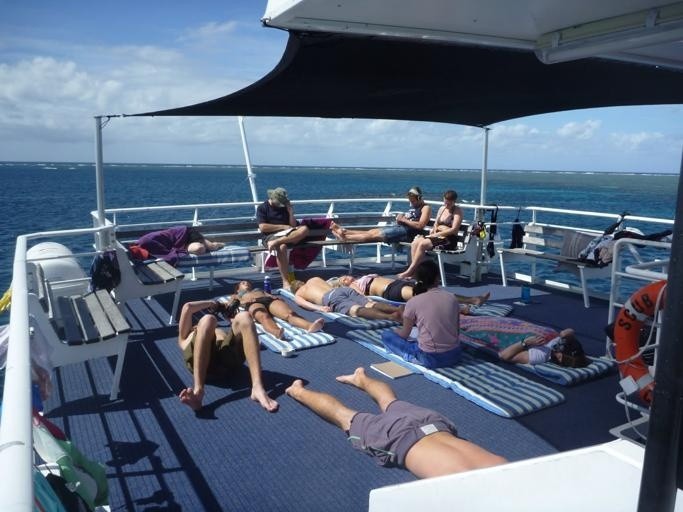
[[370, 361, 414, 380]]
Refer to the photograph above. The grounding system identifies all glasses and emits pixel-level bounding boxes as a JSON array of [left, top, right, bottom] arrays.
[[566, 350, 584, 357]]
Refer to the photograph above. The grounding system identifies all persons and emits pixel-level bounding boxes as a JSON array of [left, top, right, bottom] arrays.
[[332, 187, 433, 243], [383, 260, 461, 367], [500, 328, 585, 368], [285, 367, 512, 480], [257, 188, 309, 287], [290, 277, 404, 323], [339, 273, 491, 314], [178, 300, 279, 412], [400, 191, 463, 280], [136, 226, 225, 254], [233, 280, 325, 340]]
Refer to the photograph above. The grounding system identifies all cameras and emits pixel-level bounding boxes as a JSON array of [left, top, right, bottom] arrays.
[[220, 298, 241, 318]]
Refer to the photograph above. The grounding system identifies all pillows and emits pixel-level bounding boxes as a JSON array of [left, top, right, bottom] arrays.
[[551, 229, 596, 273]]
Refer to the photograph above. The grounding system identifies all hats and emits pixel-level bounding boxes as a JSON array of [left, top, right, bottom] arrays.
[[129, 245, 149, 260], [267, 187, 289, 204], [561, 334, 586, 367]]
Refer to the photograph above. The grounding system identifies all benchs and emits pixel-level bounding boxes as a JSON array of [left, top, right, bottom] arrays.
[[498, 222, 618, 308], [26, 240, 185, 402], [247, 211, 475, 287]]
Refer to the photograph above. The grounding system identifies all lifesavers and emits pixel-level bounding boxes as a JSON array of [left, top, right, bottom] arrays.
[[614, 280, 667, 407]]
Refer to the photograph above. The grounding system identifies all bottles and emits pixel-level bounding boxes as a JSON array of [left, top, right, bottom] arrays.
[[521, 283, 531, 304], [264, 276, 272, 295]]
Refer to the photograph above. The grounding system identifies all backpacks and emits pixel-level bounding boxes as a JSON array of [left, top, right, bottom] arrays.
[[89, 250, 121, 291], [0, 285, 109, 512]]
